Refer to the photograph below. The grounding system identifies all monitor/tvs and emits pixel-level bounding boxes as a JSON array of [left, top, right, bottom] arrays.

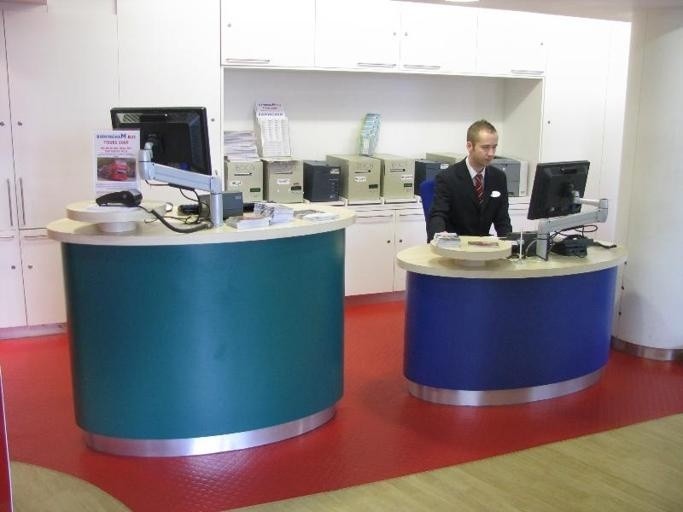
[[524, 160, 592, 221], [108, 106, 213, 190]]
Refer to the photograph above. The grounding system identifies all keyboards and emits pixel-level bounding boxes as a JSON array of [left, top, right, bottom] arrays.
[[180, 200, 279, 213]]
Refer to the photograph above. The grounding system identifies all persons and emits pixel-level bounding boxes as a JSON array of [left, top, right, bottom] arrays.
[[424, 118, 513, 244]]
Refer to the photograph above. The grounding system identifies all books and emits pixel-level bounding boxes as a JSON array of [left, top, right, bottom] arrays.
[[222, 100, 292, 164], [224, 198, 340, 232]]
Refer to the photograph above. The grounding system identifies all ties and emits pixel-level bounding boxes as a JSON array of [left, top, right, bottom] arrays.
[[474, 175, 484, 204]]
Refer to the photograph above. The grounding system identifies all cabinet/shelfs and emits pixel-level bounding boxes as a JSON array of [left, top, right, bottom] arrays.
[[504, 14, 632, 240], [344, 204, 427, 297]]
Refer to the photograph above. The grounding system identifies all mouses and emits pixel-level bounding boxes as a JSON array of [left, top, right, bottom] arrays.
[[166, 201, 174, 212]]
